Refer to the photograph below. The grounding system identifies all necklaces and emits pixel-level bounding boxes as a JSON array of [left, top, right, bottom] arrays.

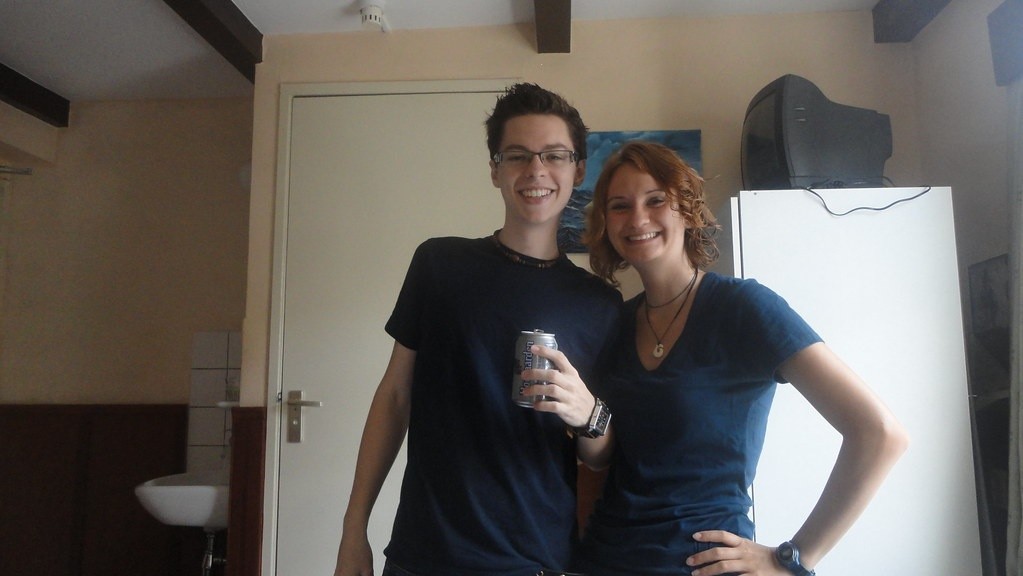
[[493, 228, 568, 269], [643, 267, 699, 357]]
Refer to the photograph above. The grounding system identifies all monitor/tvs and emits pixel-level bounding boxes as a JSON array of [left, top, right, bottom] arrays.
[[741, 74, 893, 191]]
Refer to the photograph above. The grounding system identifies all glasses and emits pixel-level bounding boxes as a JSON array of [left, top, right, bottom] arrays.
[[494, 150, 578, 168]]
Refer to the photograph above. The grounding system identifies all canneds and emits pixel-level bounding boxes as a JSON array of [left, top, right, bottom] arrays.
[[511, 329, 558, 407]]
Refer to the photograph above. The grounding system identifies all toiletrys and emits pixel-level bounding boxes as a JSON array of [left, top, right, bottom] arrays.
[[216, 454, 231, 486]]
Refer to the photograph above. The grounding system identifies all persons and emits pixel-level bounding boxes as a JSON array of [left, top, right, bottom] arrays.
[[335, 82, 624, 576], [581, 140, 906, 574]]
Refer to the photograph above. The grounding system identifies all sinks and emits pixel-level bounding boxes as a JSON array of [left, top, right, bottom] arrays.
[[135, 473, 229, 532]]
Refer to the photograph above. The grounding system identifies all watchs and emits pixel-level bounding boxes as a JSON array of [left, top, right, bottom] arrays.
[[566, 396, 611, 441], [776, 540, 816, 576]]
